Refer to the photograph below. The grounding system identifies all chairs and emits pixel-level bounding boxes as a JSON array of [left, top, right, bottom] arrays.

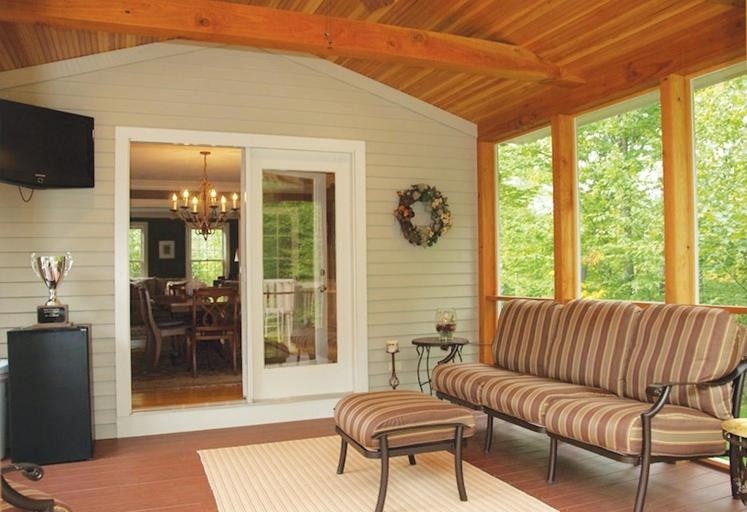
[[0, 463, 72, 512], [133, 282, 240, 378]]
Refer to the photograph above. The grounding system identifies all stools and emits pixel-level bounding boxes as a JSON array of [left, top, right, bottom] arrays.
[[334, 389, 476, 512], [721, 418, 747, 506]]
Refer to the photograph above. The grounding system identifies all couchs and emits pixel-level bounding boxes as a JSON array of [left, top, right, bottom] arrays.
[[432, 324, 747, 512]]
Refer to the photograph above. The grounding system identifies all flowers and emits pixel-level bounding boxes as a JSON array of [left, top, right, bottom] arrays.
[[393, 182, 453, 248]]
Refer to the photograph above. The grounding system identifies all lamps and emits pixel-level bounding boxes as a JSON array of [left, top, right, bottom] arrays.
[[385, 340, 400, 390], [171, 152, 239, 240]]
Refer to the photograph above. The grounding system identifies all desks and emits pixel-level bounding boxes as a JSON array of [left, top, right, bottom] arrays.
[[412, 337, 469, 398]]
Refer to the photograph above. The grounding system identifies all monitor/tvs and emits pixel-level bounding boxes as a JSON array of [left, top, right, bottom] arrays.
[[0, 98, 94, 188]]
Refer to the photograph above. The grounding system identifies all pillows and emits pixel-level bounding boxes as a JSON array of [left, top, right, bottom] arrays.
[[491, 298, 564, 380], [628, 304, 737, 421], [548, 298, 642, 398]]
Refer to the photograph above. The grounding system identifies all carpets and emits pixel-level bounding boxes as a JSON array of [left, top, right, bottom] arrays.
[[197, 434, 559, 512], [131, 325, 242, 389]]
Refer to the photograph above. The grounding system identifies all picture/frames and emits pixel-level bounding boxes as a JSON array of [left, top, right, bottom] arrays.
[[158, 240, 175, 260]]
